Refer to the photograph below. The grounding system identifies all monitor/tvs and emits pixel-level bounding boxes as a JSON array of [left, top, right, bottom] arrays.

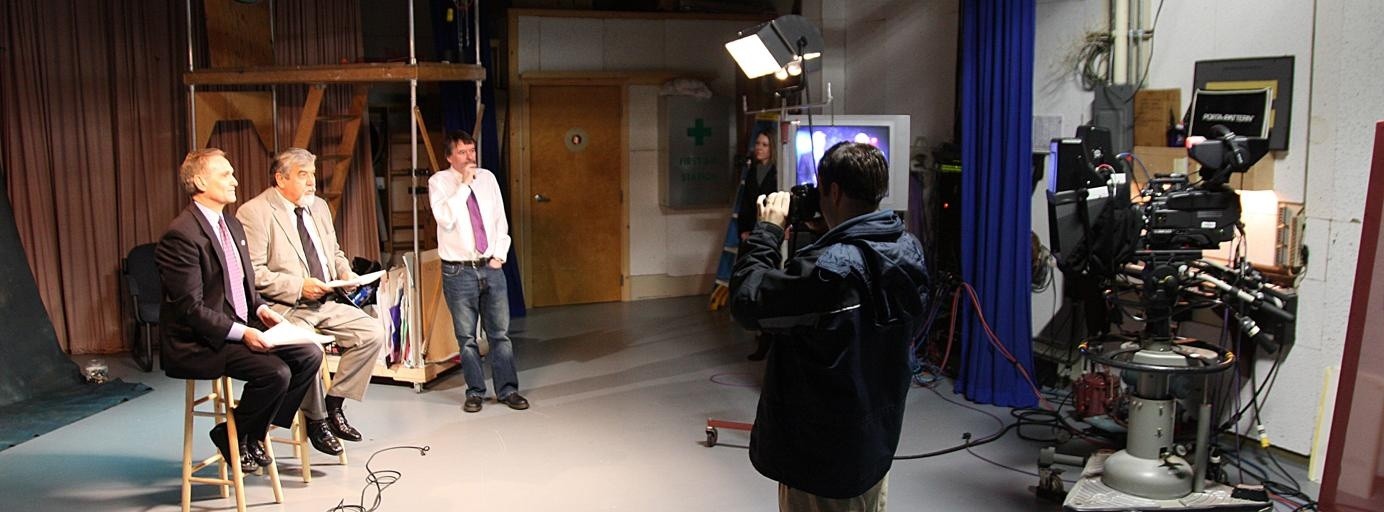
[[776, 114, 910, 211]]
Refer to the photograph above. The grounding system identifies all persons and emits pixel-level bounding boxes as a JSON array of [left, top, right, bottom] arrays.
[[727, 139, 931, 511], [426, 130, 530, 414], [151, 148, 325, 474], [798, 129, 827, 188], [737, 130, 779, 362], [235, 146, 384, 457]]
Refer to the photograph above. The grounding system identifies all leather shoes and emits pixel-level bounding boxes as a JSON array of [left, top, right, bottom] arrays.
[[306, 418, 343, 455], [209, 422, 272, 473], [497, 393, 528, 410], [464, 396, 483, 411], [326, 405, 361, 441]]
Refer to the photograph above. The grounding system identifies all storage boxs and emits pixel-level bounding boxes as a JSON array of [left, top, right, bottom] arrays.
[[1133, 88, 1274, 263], [393, 176, 431, 212], [393, 132, 442, 169], [393, 229, 427, 243]]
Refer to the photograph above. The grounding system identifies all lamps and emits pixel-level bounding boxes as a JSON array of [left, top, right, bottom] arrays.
[[725, 14, 822, 111]]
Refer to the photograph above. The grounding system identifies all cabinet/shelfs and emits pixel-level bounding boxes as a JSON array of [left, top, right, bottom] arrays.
[[1271, 201, 1304, 271], [182, 1, 487, 394], [384, 106, 448, 268]]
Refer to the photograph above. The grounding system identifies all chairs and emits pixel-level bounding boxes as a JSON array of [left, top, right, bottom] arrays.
[[121, 241, 161, 372]]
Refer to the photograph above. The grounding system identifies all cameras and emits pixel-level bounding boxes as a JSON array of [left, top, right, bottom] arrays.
[[763, 184, 820, 223]]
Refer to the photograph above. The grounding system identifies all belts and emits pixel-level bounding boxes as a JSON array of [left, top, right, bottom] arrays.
[[441, 258, 492, 266]]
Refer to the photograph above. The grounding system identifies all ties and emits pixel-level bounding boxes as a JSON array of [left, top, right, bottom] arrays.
[[466, 194, 488, 254], [218, 216, 248, 322], [294, 208, 327, 304]]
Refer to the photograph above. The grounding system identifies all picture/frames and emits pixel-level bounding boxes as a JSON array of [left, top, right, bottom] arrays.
[[1192, 56, 1295, 151]]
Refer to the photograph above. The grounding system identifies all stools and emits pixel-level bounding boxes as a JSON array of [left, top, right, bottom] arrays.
[[165, 371, 282, 512], [271, 335, 346, 485]]
